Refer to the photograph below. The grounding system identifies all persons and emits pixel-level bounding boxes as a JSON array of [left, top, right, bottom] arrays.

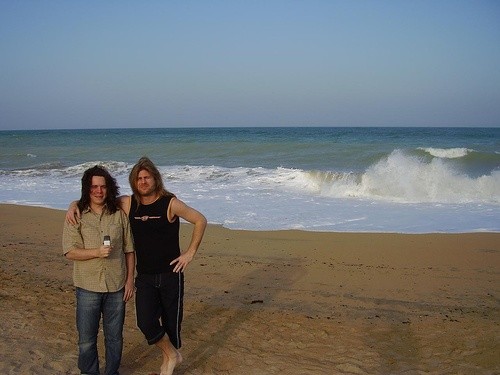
[[61, 165, 136, 375], [61, 157, 207, 375]]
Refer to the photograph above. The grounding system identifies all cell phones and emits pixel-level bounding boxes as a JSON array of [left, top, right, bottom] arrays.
[[104, 236, 110, 246]]
